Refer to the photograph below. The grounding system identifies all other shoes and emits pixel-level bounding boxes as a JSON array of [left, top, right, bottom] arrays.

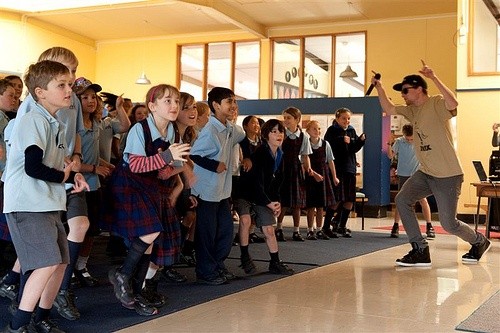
[[75, 267, 99, 287], [70, 270, 82, 289], [316, 230, 329, 240], [322, 219, 351, 239], [179, 250, 197, 267], [0, 276, 19, 299], [248, 233, 264, 243], [161, 267, 186, 283], [307, 231, 317, 240], [292, 231, 305, 241], [195, 267, 234, 285], [275, 228, 287, 241], [238, 254, 257, 274], [268, 260, 295, 275], [232, 233, 239, 246], [142, 278, 164, 306], [391, 225, 400, 238], [426, 226, 436, 240]]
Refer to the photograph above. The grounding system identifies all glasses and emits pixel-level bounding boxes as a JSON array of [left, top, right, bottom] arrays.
[[401, 86, 416, 94]]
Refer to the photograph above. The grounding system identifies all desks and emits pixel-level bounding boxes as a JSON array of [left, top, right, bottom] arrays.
[[471, 182, 500, 240]]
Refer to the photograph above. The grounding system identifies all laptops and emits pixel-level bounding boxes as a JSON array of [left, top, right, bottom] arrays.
[[473, 160, 500, 183]]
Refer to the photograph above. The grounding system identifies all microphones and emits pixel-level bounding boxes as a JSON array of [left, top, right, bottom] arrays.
[[365, 73, 381, 95]]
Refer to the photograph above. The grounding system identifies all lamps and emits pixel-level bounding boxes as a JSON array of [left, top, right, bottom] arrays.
[[340, 1, 357, 78], [136, 74, 150, 84]]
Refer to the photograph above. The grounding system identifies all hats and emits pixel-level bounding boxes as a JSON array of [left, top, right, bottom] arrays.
[[392, 74, 427, 91], [72, 76, 102, 95]]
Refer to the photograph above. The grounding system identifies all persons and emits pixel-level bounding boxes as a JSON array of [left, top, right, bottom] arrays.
[[0, 46, 435, 333], [371, 60, 491, 267]]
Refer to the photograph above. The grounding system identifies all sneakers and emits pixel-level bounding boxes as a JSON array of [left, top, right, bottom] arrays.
[[5, 322, 39, 333], [461, 234, 492, 262], [8, 301, 37, 322], [396, 242, 432, 267], [30, 317, 65, 333], [54, 288, 81, 321], [107, 264, 158, 315]]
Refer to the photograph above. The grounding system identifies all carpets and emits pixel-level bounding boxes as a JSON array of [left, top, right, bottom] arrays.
[[371, 223, 500, 240]]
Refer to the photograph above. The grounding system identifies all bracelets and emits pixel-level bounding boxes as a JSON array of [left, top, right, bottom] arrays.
[[72, 152, 82, 158], [387, 143, 391, 145], [91, 164, 96, 174]]
